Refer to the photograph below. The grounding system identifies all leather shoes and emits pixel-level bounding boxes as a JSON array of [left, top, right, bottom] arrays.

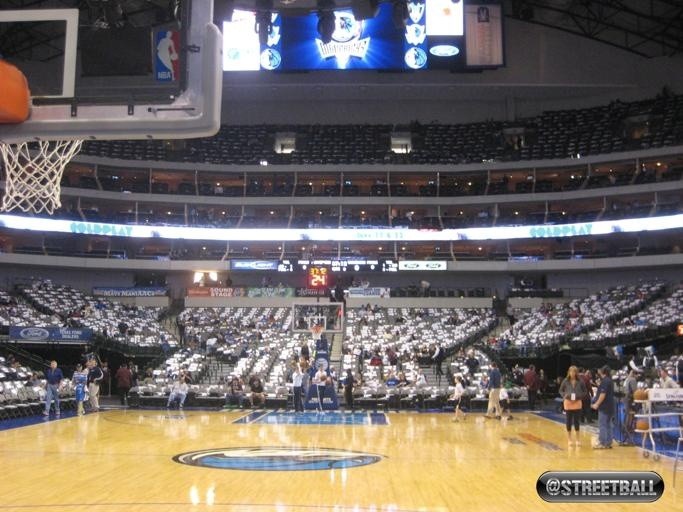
[[507, 417, 512, 420], [496, 416, 501, 419]]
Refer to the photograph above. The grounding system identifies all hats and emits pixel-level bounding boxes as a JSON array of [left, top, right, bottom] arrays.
[[76, 364, 81, 368]]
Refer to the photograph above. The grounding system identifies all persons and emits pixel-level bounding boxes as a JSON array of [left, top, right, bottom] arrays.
[[0, 85, 683, 450]]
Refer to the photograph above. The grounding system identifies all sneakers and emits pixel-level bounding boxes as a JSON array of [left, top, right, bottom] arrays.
[[593, 443, 605, 449], [463, 413, 467, 420]]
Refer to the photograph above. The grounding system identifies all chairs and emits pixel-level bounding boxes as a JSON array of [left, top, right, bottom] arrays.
[[1, 96, 681, 261], [1, 282, 683, 430]]
